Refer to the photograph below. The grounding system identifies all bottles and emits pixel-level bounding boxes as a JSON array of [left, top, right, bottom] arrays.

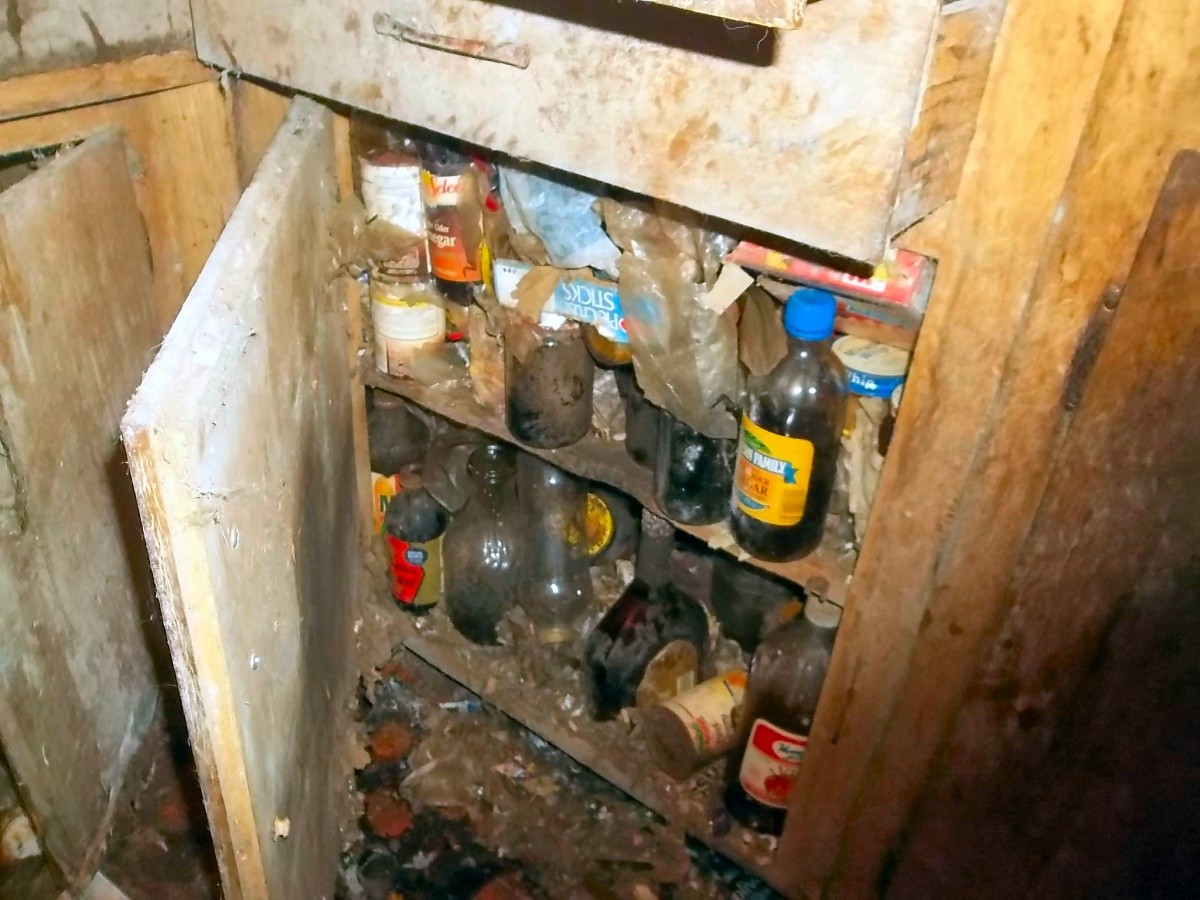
[[344, 106, 910, 836]]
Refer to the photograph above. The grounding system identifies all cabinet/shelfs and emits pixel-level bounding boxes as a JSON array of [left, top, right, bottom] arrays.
[[2, 1, 1000, 900]]
